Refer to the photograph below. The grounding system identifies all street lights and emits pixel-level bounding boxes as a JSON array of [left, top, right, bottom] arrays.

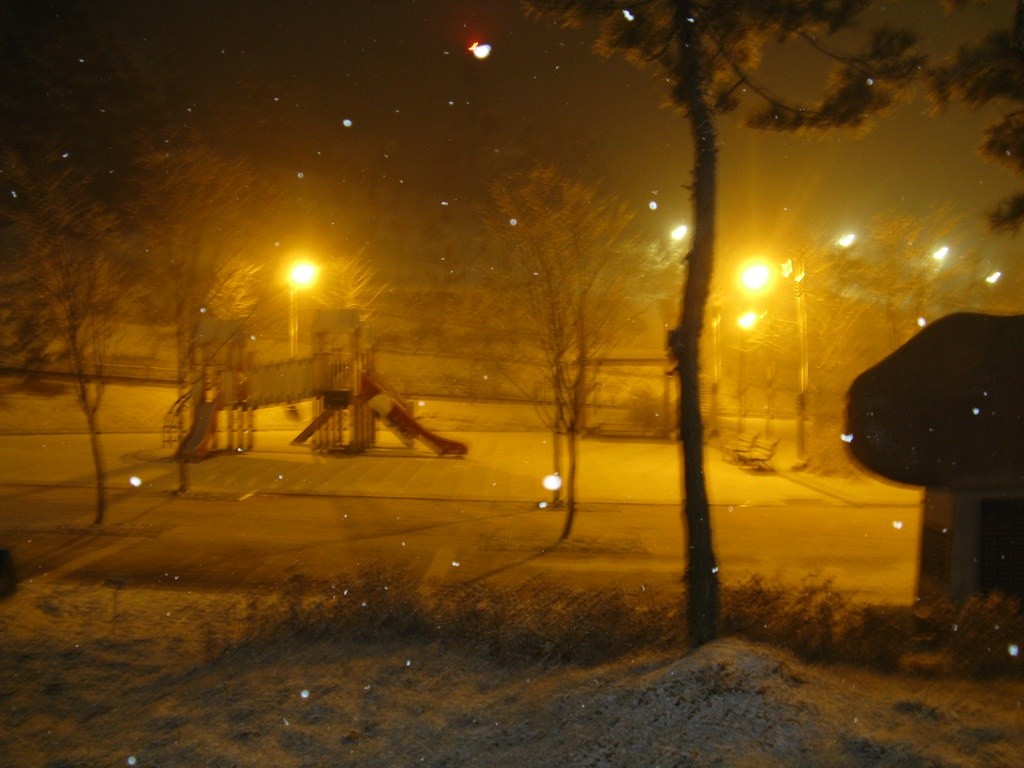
[[736, 264, 769, 443], [796, 234, 859, 458], [662, 219, 691, 429], [285, 262, 316, 361], [910, 253, 952, 338]]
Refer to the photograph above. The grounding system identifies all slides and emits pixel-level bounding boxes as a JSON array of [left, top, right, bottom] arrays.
[[360, 372, 470, 455]]
